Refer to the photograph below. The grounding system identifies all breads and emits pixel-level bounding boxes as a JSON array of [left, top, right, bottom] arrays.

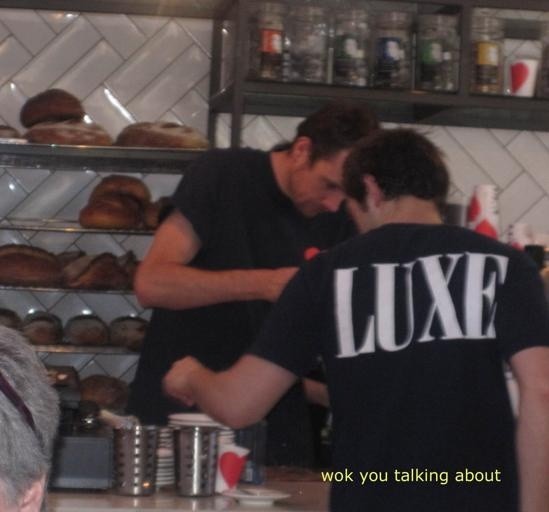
[[0, 88, 212, 406]]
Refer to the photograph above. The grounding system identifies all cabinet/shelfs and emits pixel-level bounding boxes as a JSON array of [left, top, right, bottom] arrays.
[[2, 1, 218, 436], [210, 0, 549, 132]]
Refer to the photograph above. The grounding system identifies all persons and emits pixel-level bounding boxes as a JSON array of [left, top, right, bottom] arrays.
[[126, 102, 549, 512], [0, 324, 60, 512]]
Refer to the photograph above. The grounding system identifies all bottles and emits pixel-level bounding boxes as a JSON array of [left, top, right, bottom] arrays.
[[245, 0, 462, 93], [464, 7, 506, 95]]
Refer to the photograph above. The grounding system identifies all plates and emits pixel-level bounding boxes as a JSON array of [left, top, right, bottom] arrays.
[[153, 413, 235, 485], [222, 488, 291, 505]]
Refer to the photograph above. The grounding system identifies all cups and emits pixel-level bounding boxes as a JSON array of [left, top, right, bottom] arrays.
[[467, 185, 501, 239], [507, 222, 534, 251], [215, 443, 249, 494], [510, 58, 538, 98]]
